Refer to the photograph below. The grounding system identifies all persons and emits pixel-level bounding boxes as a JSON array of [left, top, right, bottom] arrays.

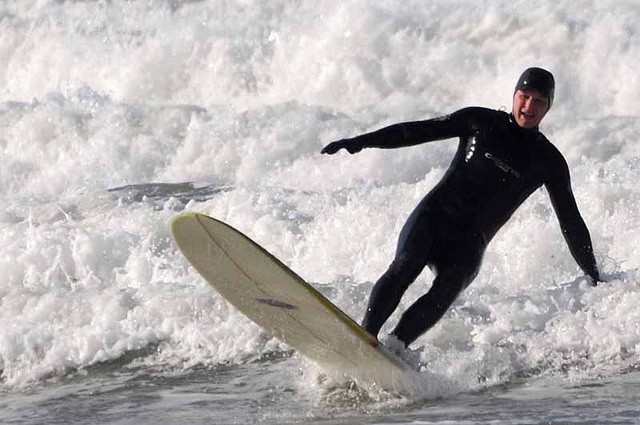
[[321, 67, 607, 347]]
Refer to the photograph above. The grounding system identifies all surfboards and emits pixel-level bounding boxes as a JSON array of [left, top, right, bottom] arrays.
[[170, 212, 415, 389]]
